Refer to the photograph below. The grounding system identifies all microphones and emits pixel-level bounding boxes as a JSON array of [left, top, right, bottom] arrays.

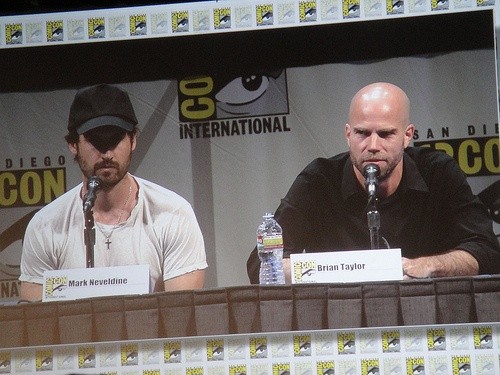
[[83, 176, 103, 213], [363, 163, 381, 204]]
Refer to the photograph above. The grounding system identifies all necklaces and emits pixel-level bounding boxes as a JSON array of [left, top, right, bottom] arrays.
[[81, 178, 132, 249]]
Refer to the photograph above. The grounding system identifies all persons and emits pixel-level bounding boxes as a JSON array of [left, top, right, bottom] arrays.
[[247, 82, 500, 285], [19, 84, 208, 304]]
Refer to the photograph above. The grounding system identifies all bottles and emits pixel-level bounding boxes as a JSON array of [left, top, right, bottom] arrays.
[[256, 211, 285, 284]]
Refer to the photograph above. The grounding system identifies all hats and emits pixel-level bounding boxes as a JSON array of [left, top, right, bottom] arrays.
[[67, 83, 138, 134]]
